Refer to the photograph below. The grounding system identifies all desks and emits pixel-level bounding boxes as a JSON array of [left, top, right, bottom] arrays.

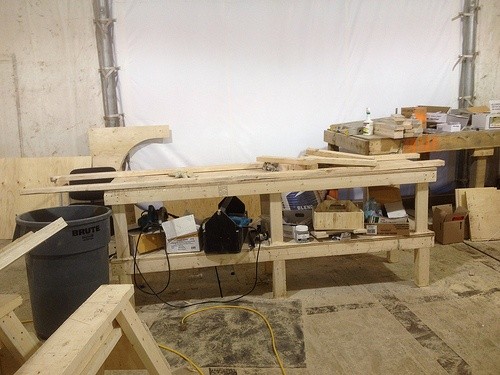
[[104, 165, 438, 310], [324, 127, 500, 232]]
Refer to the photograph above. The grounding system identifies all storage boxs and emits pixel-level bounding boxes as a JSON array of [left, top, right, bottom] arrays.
[[432, 206, 471, 245], [202, 195, 250, 254], [128, 214, 201, 254], [401, 99, 500, 133], [312, 199, 365, 229], [282, 189, 328, 208]]
